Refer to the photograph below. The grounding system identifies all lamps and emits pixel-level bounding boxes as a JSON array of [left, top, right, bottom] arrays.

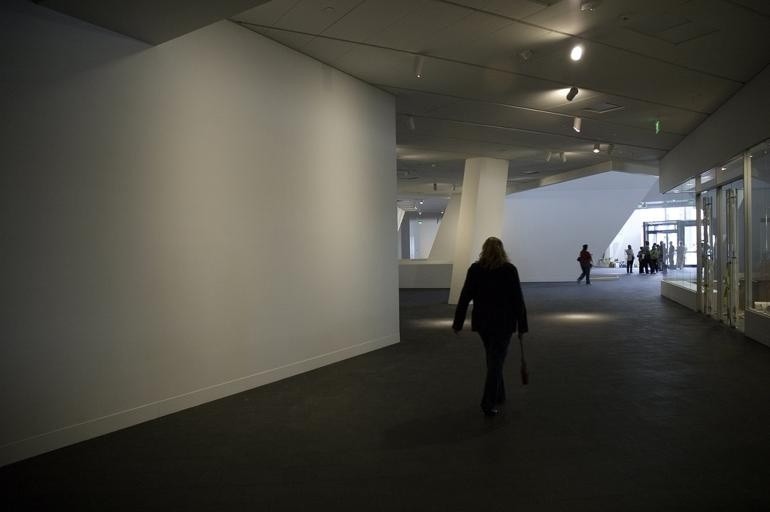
[[415, 56, 424, 80], [593, 142, 600, 155], [519, 48, 534, 62], [409, 116, 417, 130], [573, 115, 582, 134], [559, 152, 566, 164], [544, 151, 552, 162], [566, 87, 578, 101]]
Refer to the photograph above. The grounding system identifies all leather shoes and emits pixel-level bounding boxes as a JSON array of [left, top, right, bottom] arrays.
[[480, 404, 504, 416]]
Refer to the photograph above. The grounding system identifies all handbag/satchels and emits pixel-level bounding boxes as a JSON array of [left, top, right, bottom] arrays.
[[522, 361, 529, 385]]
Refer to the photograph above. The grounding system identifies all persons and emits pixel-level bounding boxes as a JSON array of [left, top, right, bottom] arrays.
[[577, 245, 594, 286], [625, 245, 635, 273], [636, 240, 688, 274], [452, 237, 529, 421]]
